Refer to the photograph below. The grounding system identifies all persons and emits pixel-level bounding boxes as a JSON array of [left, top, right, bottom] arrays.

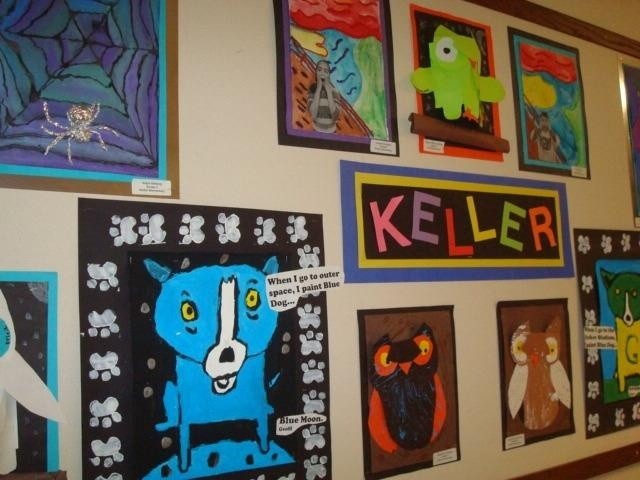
[[529, 112, 560, 163], [307, 60, 340, 133]]
